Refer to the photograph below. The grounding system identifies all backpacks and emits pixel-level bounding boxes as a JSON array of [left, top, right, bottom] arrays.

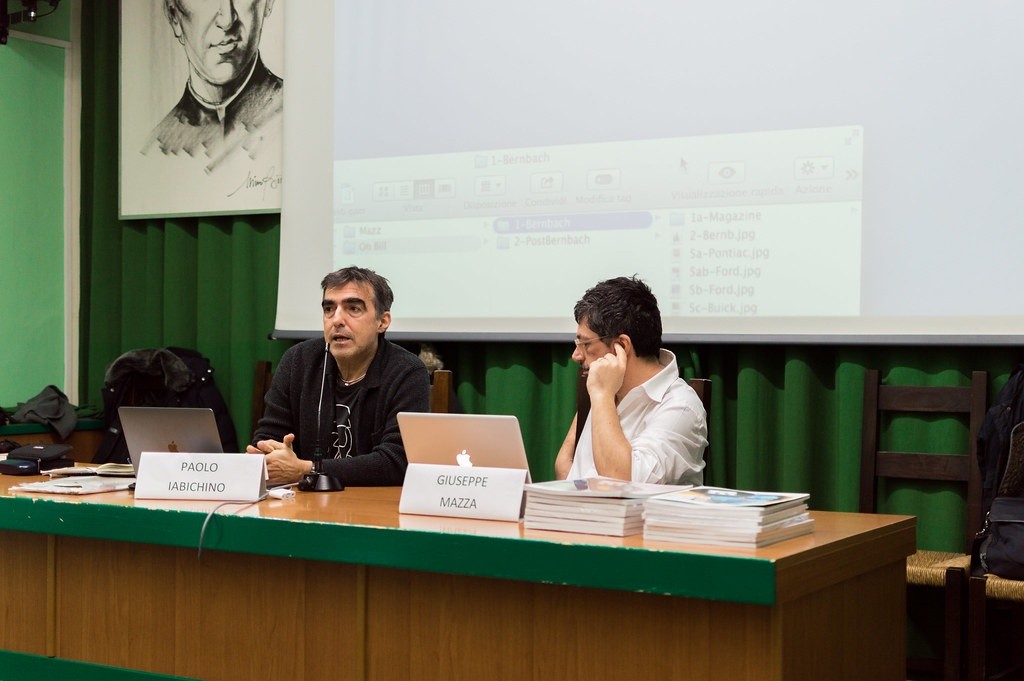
[[969, 367, 1024, 581]]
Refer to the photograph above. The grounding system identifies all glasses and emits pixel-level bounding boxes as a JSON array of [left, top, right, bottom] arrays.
[[573, 334, 611, 352]]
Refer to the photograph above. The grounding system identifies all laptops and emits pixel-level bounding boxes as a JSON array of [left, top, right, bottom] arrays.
[[396, 412, 533, 483], [118, 406, 224, 479]]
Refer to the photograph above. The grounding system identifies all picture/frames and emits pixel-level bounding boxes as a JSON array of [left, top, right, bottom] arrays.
[[118, 0, 283, 220]]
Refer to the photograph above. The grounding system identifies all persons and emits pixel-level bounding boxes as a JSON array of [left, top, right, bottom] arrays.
[[554, 274, 709, 488], [245, 267, 430, 488]]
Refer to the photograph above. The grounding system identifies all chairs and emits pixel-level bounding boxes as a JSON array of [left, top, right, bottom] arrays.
[[966, 520, 1024, 681], [247, 357, 280, 449], [574, 371, 713, 491], [859, 368, 989, 681], [418, 364, 457, 413]]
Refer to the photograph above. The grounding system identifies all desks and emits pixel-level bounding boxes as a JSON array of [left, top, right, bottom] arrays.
[[3, 440, 917, 681]]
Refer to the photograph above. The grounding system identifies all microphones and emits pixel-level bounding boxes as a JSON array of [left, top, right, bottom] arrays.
[[297, 342, 343, 492]]
